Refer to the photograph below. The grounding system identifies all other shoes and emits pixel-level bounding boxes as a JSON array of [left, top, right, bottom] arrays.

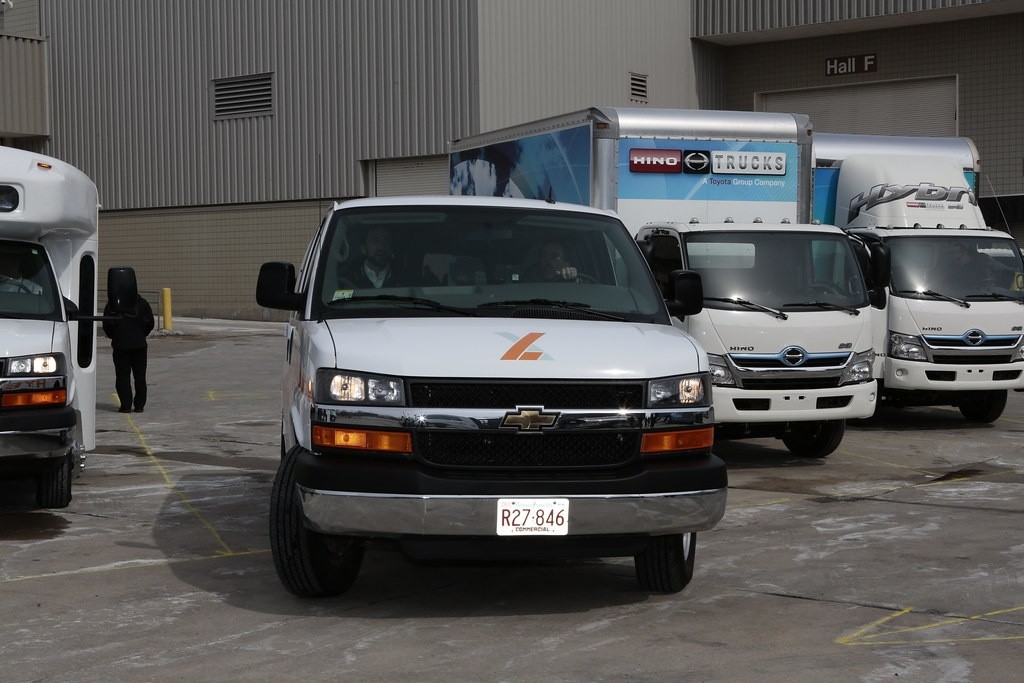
[[133, 407, 144, 412], [119, 408, 132, 413]]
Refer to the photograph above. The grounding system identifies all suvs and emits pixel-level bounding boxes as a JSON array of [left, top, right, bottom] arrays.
[[256, 194, 728, 601]]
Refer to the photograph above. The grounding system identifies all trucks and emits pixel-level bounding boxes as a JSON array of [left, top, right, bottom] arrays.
[[446, 106, 887, 458], [809, 131, 1024, 423], [0, 145, 139, 512]]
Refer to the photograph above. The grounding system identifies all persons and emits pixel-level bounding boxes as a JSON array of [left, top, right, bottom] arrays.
[[336, 223, 419, 303], [103, 279, 155, 413], [0, 254, 42, 294], [509, 240, 585, 287]]
